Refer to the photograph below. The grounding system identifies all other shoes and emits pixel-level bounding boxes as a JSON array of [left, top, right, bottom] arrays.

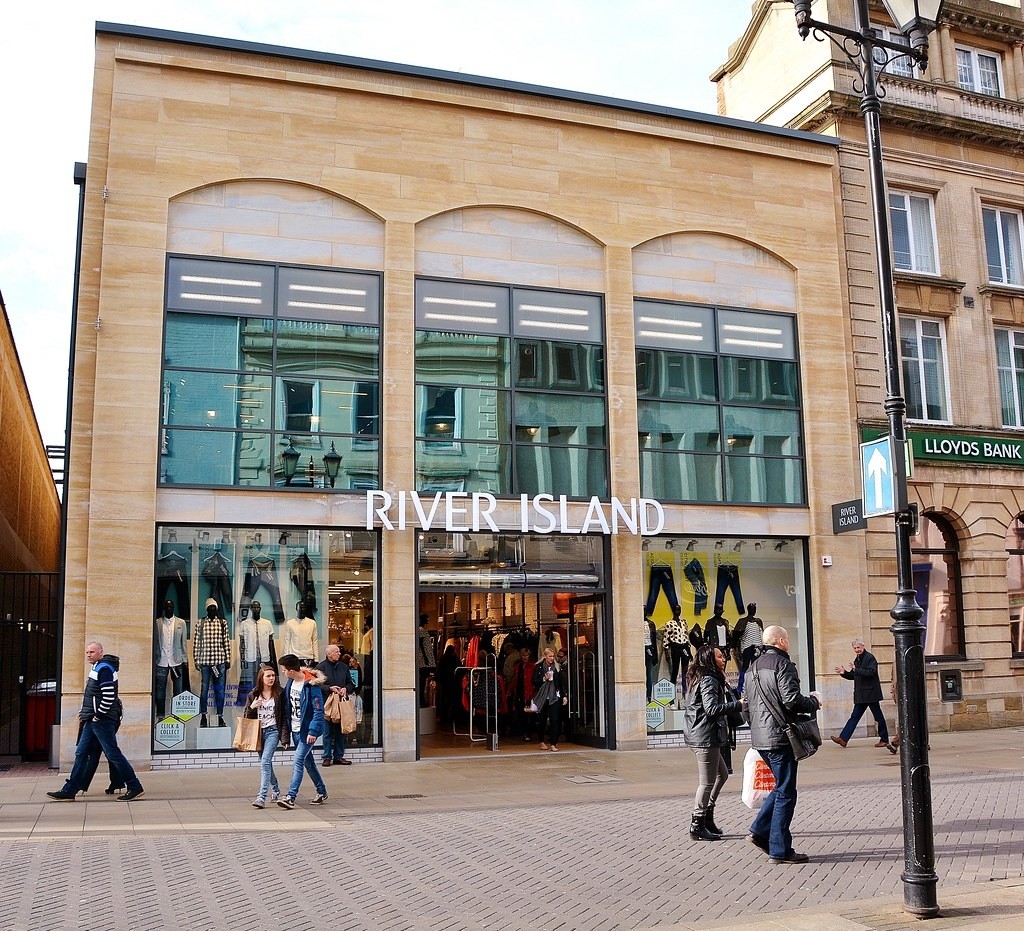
[[538, 742, 548, 750], [887, 743, 897, 754], [875, 742, 889, 747], [219, 717, 226, 727], [201, 719, 207, 727], [551, 745, 558, 752], [524, 734, 531, 742], [831, 735, 847, 747]]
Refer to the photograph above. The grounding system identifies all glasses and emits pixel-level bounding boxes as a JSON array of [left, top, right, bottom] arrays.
[[556, 655, 565, 658]]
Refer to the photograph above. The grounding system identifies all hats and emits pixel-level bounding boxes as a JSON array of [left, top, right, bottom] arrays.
[[206, 598, 218, 610]]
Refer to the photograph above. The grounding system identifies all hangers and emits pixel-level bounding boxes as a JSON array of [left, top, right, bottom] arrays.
[[247, 551, 275, 566], [204, 550, 231, 563], [291, 553, 317, 565], [718, 557, 739, 568], [685, 555, 704, 568], [651, 557, 671, 567], [452, 625, 553, 636], [160, 548, 189, 562]]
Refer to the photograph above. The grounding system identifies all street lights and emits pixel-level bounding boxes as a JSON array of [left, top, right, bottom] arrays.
[[794, 0, 942, 916]]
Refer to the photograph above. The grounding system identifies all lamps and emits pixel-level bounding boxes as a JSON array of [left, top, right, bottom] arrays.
[[641, 538, 789, 553], [168, 528, 321, 547]]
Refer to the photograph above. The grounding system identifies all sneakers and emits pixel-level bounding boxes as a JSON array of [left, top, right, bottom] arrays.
[[746, 832, 769, 854], [769, 852, 809, 864], [116, 787, 145, 801], [270, 792, 280, 802], [46, 791, 74, 801], [252, 797, 266, 809], [277, 795, 294, 809], [310, 793, 328, 805]]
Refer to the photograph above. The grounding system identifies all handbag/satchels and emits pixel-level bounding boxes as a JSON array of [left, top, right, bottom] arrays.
[[324, 692, 357, 734], [783, 717, 823, 760], [742, 748, 777, 810], [233, 714, 262, 752]]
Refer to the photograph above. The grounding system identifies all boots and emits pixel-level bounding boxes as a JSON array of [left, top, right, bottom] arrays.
[[690, 814, 721, 841], [705, 806, 723, 834]]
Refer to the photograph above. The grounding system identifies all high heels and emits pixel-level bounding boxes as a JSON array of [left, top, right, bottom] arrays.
[[105, 786, 121, 794], [76, 789, 85, 795]]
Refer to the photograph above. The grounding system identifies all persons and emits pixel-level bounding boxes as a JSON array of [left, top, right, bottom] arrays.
[[285, 601, 319, 668], [435, 645, 571, 752], [193, 598, 231, 726], [643, 604, 659, 703], [663, 605, 693, 710], [743, 626, 822, 863], [46, 642, 146, 801], [317, 645, 354, 766], [243, 667, 290, 809], [277, 654, 328, 808], [703, 604, 731, 673], [884, 662, 930, 754], [830, 640, 889, 747], [342, 654, 362, 691], [552, 593, 577, 619], [733, 603, 764, 697], [154, 601, 188, 722], [682, 646, 744, 840], [239, 601, 277, 695], [419, 615, 435, 707]]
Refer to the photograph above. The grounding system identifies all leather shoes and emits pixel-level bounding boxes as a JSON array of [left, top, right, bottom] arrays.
[[322, 759, 330, 766], [333, 758, 351, 765]]
